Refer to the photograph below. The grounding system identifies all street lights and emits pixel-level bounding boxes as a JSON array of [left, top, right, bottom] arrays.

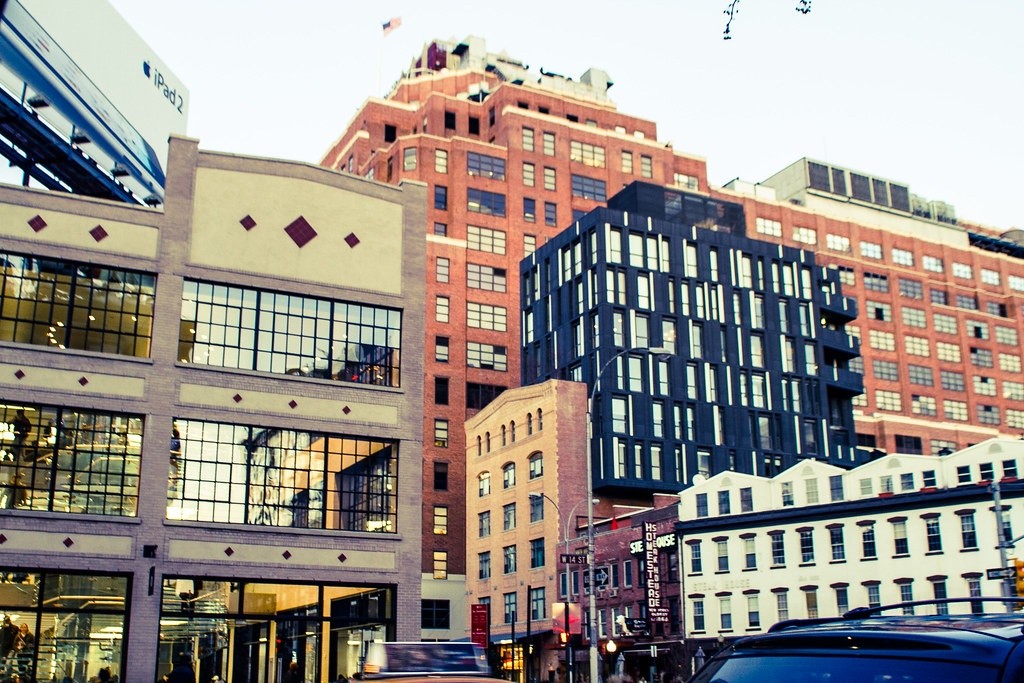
[[529, 492, 600, 683], [587, 346, 676, 683]]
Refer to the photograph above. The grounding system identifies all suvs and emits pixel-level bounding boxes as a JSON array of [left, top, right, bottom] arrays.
[[681, 596, 1024, 683]]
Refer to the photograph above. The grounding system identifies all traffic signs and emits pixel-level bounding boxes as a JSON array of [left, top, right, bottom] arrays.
[[988, 566, 1017, 580]]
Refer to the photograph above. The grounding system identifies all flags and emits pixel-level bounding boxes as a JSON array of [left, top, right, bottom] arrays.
[[381, 15, 402, 35]]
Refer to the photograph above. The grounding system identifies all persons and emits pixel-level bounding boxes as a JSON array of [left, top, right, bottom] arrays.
[[7, 408, 32, 444], [0, 616, 35, 671], [0, 473, 28, 509]]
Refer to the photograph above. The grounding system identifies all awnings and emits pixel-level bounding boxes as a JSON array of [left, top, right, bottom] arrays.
[[449, 629, 552, 646]]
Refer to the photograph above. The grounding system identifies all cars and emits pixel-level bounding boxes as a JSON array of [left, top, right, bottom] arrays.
[[346, 639, 515, 683]]
